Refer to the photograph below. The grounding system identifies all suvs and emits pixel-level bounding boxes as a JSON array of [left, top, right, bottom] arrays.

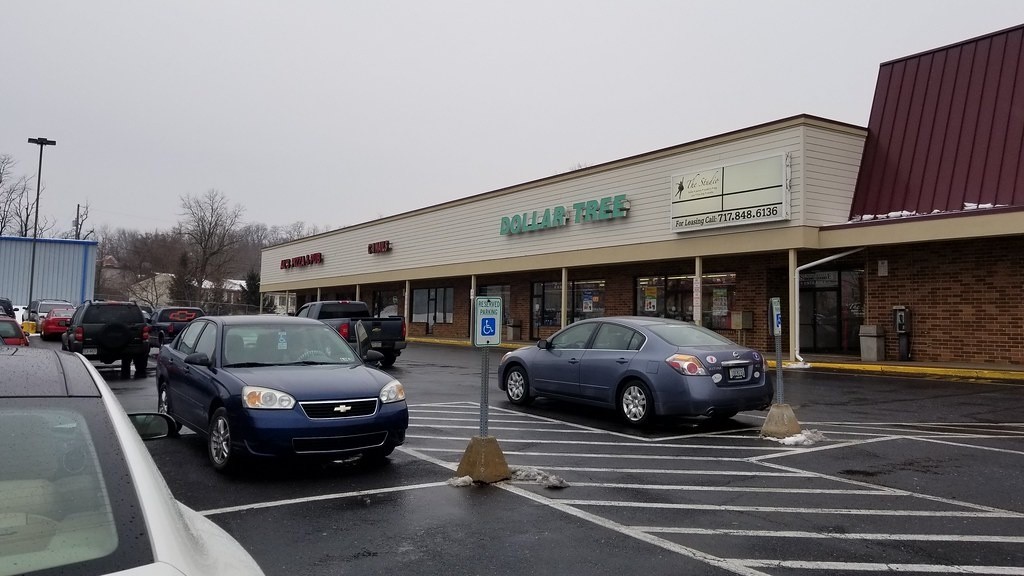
[[63, 298, 154, 382]]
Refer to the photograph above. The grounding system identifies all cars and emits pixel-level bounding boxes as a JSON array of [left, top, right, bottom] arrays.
[[154, 313, 410, 472], [0, 315, 32, 347], [0, 297, 20, 320], [495, 314, 775, 434], [39, 308, 75, 340], [0, 344, 271, 575], [21, 297, 72, 334], [148, 304, 206, 348]]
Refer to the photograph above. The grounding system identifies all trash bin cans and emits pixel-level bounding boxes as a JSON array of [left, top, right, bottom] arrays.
[[858, 324, 885, 362]]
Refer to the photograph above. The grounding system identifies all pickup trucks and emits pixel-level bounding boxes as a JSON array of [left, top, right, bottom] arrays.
[[291, 298, 409, 371]]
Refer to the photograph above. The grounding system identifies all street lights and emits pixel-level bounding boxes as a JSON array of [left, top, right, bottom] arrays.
[[25, 135, 56, 304]]
